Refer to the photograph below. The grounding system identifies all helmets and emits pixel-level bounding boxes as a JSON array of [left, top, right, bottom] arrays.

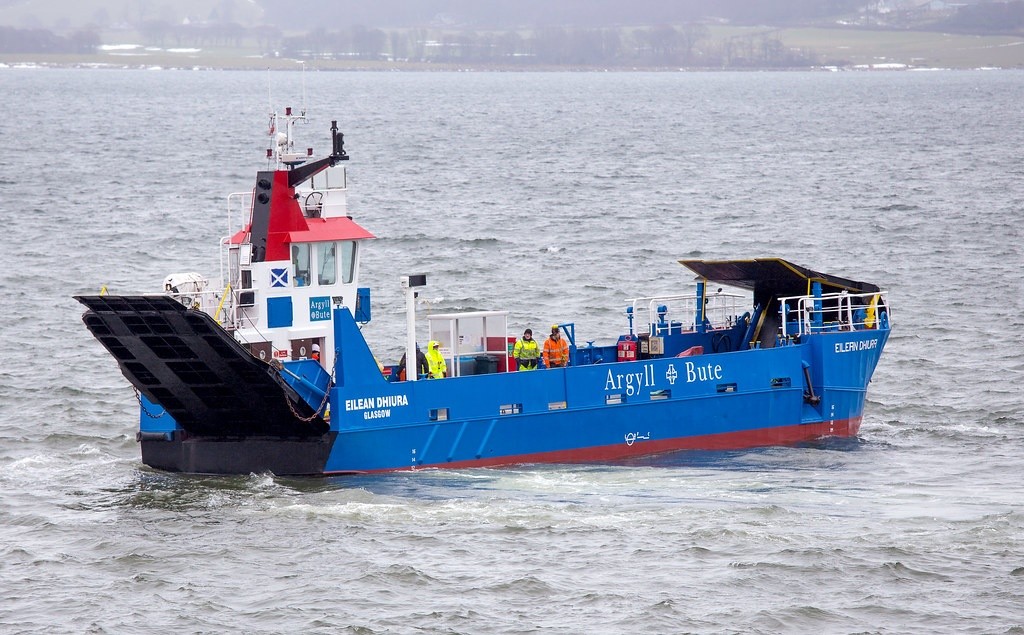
[[312, 344, 321, 352]]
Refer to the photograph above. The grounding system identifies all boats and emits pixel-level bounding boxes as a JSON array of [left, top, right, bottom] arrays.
[[72, 108, 900, 479]]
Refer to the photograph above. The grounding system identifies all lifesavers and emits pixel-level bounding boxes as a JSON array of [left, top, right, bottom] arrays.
[[880, 311, 888, 328]]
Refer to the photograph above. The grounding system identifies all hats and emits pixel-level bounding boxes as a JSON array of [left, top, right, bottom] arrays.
[[433, 343, 439, 347], [524, 329, 532, 336]]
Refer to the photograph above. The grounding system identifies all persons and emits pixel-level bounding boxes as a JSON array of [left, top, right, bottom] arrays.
[[311, 343, 320, 362], [395, 342, 428, 381], [422, 341, 447, 379], [543, 324, 569, 369], [512, 329, 540, 371]]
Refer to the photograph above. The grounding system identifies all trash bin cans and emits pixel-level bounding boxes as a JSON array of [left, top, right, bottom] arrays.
[[474, 355, 500, 375]]
